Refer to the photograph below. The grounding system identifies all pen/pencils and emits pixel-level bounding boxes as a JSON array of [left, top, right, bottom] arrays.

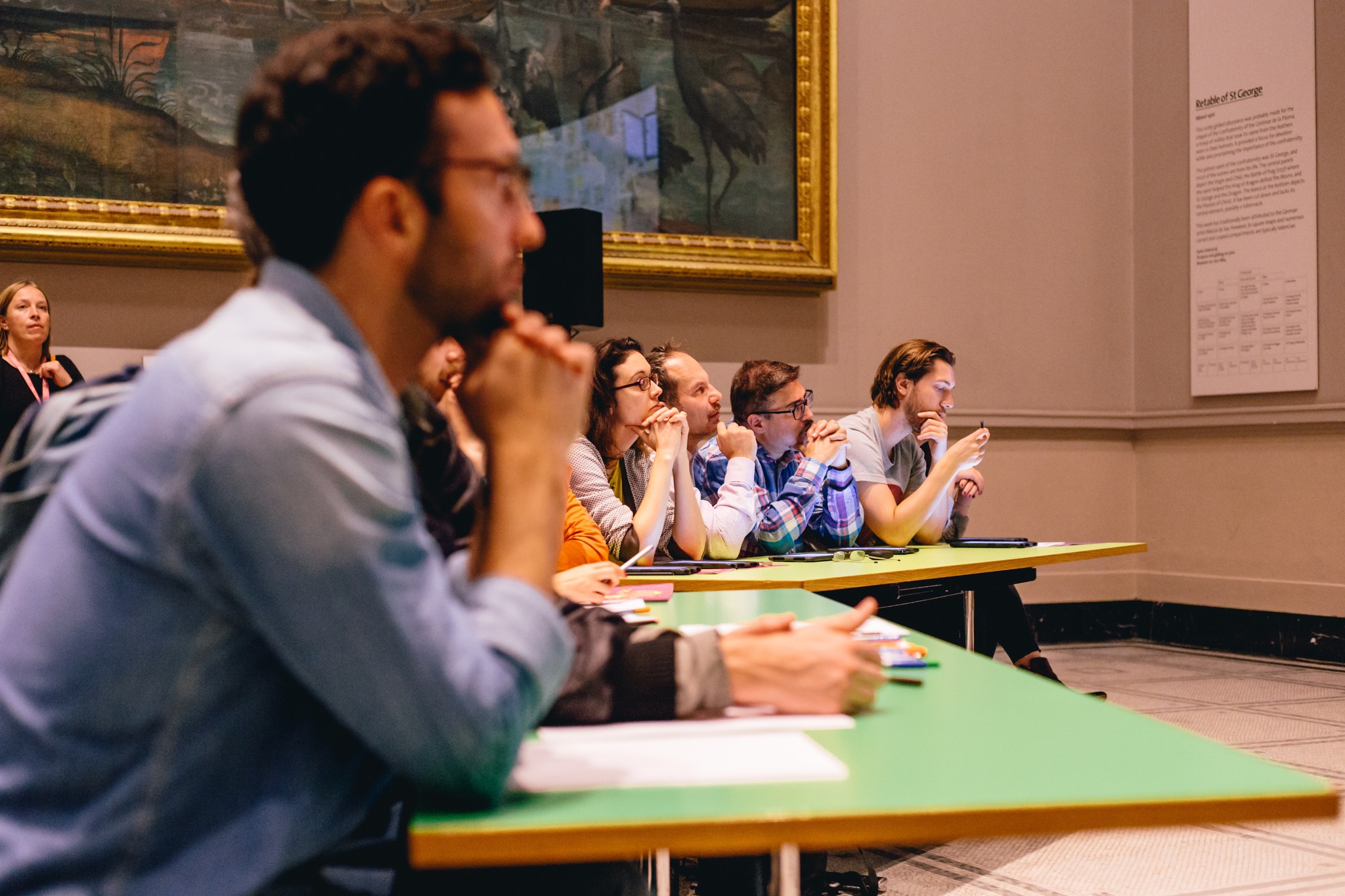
[[890, 677, 922, 685], [981, 420, 984, 428], [617, 605, 652, 616], [892, 661, 940, 669]]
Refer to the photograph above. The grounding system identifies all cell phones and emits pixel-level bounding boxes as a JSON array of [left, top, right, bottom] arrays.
[[945, 536, 1028, 543], [949, 541, 1038, 548], [827, 547, 919, 555], [653, 559, 759, 569], [768, 552, 835, 562], [628, 565, 701, 575]]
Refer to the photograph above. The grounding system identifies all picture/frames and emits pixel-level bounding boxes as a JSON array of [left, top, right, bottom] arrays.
[[0, 0, 839, 294]]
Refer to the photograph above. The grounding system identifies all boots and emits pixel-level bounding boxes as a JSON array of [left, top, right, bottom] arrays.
[[1019, 657, 1107, 701]]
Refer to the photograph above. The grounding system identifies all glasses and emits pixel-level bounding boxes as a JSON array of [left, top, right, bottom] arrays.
[[832, 550, 901, 563], [418, 153, 531, 207], [613, 373, 658, 391], [747, 390, 814, 420]]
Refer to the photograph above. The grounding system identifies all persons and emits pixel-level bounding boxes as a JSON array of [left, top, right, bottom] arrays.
[[0, 9, 594, 896], [1, 278, 1109, 729]]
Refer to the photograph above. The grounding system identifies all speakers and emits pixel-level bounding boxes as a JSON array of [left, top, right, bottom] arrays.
[[523, 208, 603, 332]]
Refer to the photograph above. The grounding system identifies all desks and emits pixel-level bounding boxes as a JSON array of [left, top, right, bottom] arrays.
[[619, 542, 1148, 654], [406, 588, 1341, 896]]
